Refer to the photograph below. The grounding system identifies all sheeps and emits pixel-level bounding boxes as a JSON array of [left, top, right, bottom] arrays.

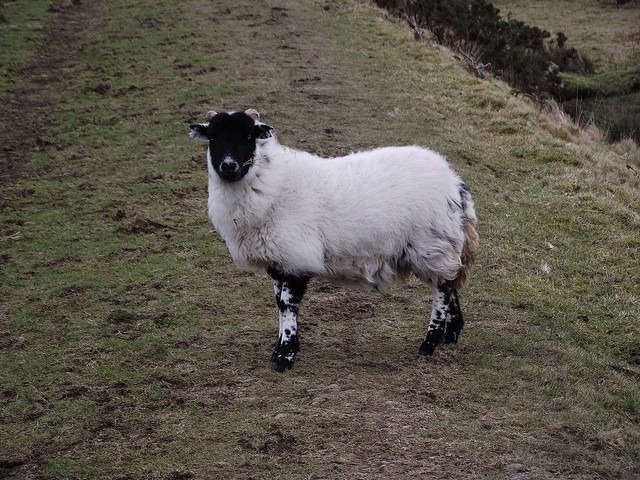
[[189, 109, 479, 372]]
